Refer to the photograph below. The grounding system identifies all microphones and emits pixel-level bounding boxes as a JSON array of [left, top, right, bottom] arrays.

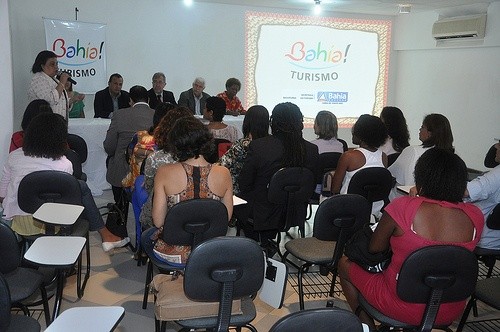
[[56, 71, 77, 85]]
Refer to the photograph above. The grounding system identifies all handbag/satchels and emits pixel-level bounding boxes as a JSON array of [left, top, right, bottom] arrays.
[[344, 223, 390, 273], [96, 202, 125, 237]]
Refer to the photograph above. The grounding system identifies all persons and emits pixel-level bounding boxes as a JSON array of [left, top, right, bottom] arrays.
[[103, 85, 233, 274], [380, 106, 410, 162], [30, 50, 85, 123], [339, 147, 484, 332], [409, 139, 500, 254], [204, 96, 243, 141], [331, 114, 389, 223], [214, 102, 320, 258], [387, 113, 455, 201], [56, 71, 85, 118], [217, 78, 246, 116], [94, 73, 131, 118], [311, 111, 343, 200], [9, 98, 53, 151], [147, 72, 176, 109], [178, 77, 211, 115], [0, 113, 130, 251]]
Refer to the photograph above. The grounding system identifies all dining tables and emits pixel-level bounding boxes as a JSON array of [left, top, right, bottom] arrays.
[[161, 236, 266, 332]]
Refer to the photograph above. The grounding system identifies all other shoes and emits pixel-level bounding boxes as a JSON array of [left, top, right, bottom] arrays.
[[102, 237, 131, 252]]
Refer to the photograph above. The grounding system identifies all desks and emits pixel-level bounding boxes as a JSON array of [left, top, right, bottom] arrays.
[[396, 183, 420, 199], [44, 303, 125, 332], [261, 254, 289, 309], [69, 114, 244, 194], [22, 232, 89, 269], [32, 201, 86, 233]]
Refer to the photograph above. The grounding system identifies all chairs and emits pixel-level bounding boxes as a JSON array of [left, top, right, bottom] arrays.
[[355, 242, 479, 332], [69, 132, 89, 164], [236, 166, 321, 258], [283, 191, 372, 312], [475, 202, 500, 280], [0, 168, 92, 332], [347, 165, 396, 210], [268, 308, 364, 332], [458, 273, 500, 332], [141, 199, 230, 309]]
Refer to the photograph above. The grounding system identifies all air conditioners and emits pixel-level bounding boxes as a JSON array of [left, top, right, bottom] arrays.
[[430, 13, 487, 43]]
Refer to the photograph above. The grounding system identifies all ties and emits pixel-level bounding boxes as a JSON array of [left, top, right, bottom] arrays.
[[157, 94, 161, 103]]
